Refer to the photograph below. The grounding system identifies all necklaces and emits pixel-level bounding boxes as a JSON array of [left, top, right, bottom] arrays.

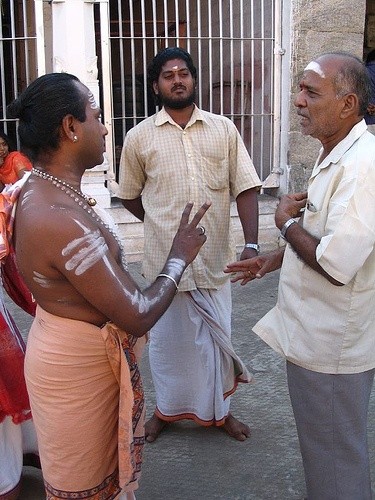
[[31, 168, 128, 270]]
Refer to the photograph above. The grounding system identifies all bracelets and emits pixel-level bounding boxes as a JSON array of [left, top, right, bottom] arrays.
[[246, 244, 260, 252], [281, 218, 295, 237], [158, 274, 179, 295]]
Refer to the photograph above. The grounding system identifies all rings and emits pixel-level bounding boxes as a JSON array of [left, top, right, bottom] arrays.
[[200, 226, 205, 234]]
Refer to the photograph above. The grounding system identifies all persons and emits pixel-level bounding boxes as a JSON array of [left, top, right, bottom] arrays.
[[223, 51, 375, 500], [115, 47, 263, 442], [0, 73, 212, 500], [0, 133, 33, 195]]
